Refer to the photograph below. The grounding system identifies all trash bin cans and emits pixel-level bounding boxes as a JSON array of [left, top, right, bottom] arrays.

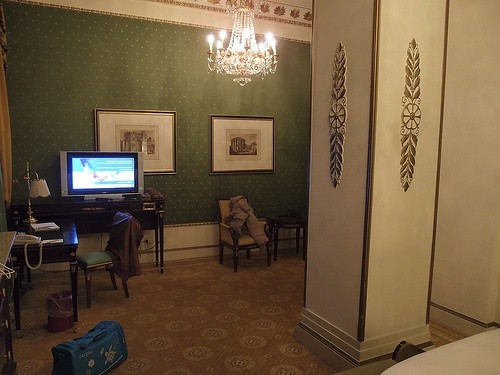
[[46, 290, 73, 333]]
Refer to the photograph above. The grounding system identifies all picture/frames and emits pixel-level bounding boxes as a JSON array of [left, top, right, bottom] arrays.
[[209, 114, 276, 175], [93, 107, 178, 175]]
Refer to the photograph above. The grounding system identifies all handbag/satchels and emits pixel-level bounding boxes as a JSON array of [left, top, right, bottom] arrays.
[[51, 320, 128, 375]]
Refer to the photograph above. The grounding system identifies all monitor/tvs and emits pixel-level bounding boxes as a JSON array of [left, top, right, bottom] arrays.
[[60, 151, 144, 199]]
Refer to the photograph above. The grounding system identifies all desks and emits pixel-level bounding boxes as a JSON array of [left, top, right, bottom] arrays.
[[265, 211, 308, 262], [10, 199, 166, 290], [10, 223, 79, 339]]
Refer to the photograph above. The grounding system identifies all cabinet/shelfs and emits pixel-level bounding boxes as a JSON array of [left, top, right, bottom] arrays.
[[0, 231, 17, 375]]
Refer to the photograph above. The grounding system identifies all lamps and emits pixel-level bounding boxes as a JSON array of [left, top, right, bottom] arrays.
[[206, 0, 280, 86], [12, 162, 51, 223]]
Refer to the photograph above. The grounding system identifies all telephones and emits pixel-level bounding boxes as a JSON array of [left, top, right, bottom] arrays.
[[12, 233, 41, 245]]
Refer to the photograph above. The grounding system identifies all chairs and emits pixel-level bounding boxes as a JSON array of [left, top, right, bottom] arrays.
[[215, 198, 272, 273], [76, 212, 145, 308]]
